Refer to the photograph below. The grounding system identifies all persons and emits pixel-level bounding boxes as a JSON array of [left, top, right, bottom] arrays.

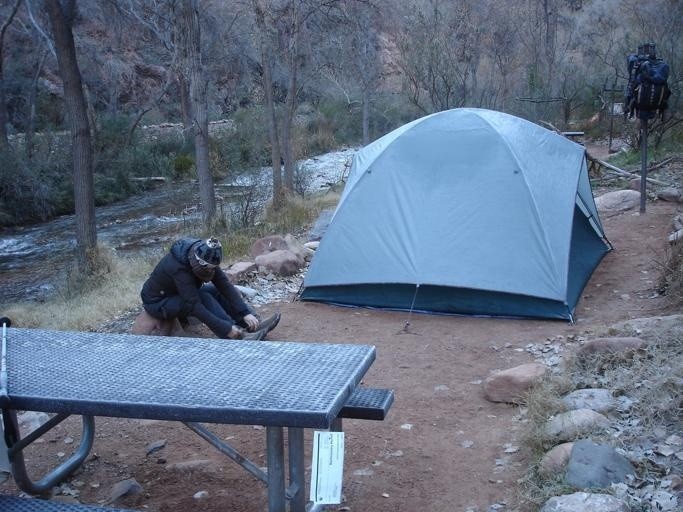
[[140, 237, 281, 341]]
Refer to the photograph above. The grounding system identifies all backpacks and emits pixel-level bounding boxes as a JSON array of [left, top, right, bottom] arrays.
[[624, 54, 671, 121]]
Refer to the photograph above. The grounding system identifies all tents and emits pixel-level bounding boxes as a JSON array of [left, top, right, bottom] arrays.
[[295, 108, 612, 322]]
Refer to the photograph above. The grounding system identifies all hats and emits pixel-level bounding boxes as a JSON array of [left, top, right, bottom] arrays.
[[194, 236, 223, 267]]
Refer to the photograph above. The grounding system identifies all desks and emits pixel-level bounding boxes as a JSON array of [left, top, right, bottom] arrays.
[[0, 324, 376, 507]]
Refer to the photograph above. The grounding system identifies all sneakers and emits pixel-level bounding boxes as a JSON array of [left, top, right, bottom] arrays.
[[240, 328, 266, 340], [257, 313, 281, 332]]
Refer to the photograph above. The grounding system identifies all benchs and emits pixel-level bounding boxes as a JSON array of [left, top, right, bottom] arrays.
[[342, 385, 396, 420]]
